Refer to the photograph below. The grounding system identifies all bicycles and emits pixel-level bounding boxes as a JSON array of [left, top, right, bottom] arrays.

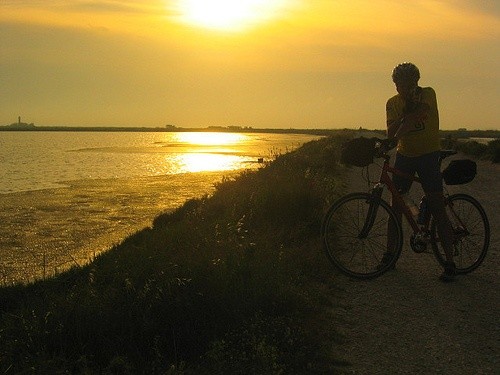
[[321, 135, 490, 279]]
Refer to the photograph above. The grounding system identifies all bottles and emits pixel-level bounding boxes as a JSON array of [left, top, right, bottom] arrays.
[[418, 197, 426, 224]]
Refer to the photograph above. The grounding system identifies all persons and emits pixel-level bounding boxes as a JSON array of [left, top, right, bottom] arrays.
[[376, 62, 458, 282]]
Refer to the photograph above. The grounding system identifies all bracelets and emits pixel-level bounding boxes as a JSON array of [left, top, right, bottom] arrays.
[[400, 116, 406, 123]]
